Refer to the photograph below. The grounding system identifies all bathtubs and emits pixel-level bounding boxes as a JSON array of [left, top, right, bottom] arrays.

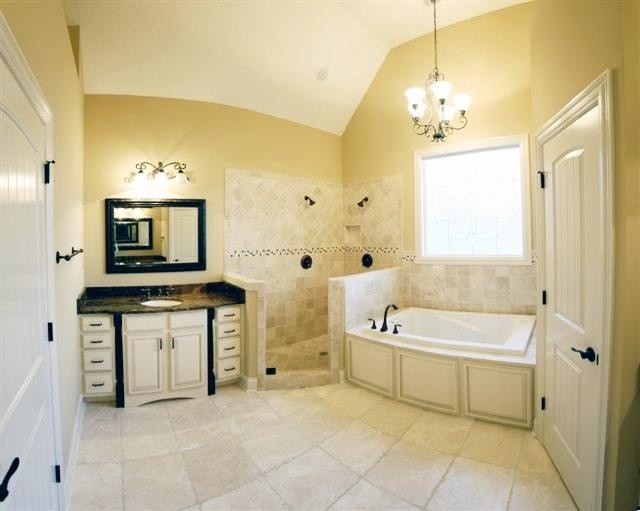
[[363, 308, 536, 355]]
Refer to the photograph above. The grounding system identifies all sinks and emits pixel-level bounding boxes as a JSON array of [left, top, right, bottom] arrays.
[[140, 298, 184, 307]]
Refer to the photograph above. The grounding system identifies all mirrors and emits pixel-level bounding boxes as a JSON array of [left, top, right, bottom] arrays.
[[104, 197, 207, 274], [113, 215, 153, 251]]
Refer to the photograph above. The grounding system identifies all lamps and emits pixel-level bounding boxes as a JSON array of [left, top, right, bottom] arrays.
[[405, 1, 468, 144], [124, 162, 191, 196]]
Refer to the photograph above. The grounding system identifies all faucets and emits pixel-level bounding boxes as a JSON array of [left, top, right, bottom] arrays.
[[156, 287, 162, 296], [380, 304, 397, 332]]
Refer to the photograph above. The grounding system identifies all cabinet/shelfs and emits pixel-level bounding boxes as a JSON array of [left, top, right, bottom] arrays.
[[78, 312, 116, 398], [214, 307, 243, 381], [121, 309, 207, 397]]
